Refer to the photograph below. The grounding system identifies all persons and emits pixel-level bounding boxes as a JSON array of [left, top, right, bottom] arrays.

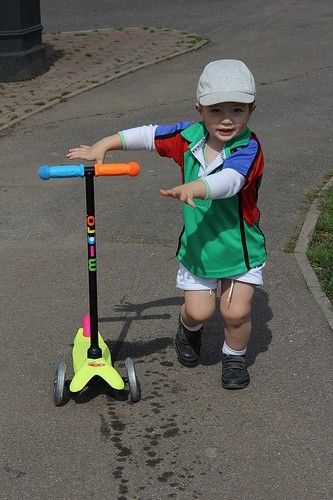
[[66, 58, 269, 390]]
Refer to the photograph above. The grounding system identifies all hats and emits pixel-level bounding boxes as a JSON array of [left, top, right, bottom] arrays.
[[196, 59, 256, 107]]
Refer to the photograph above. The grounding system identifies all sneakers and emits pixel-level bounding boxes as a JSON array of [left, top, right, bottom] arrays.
[[174, 312, 203, 363], [221, 350, 249, 389]]
